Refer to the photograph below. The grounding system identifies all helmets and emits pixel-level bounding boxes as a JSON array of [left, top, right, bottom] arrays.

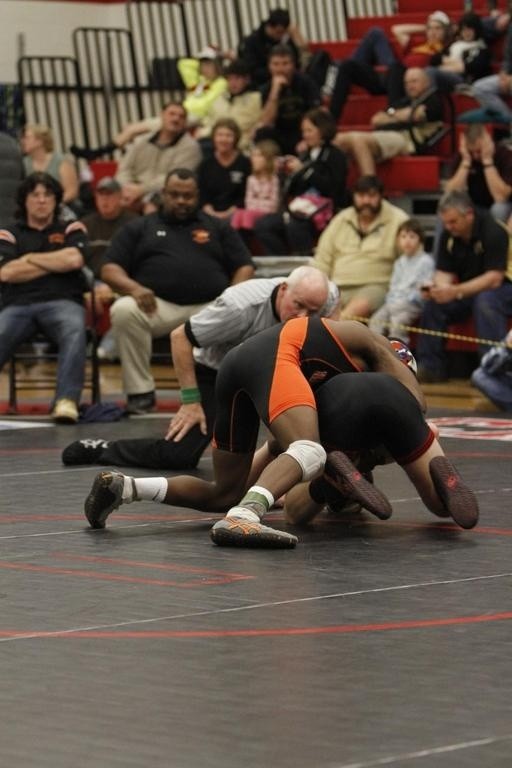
[[387, 336, 418, 375]]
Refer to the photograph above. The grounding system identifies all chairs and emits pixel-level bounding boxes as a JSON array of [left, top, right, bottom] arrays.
[[7, 273, 101, 411]]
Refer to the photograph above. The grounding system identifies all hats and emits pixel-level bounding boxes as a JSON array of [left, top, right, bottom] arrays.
[[427, 9, 449, 25], [192, 44, 217, 60], [94, 176, 120, 192]]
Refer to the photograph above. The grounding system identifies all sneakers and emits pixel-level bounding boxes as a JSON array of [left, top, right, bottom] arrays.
[[61, 437, 109, 461], [321, 450, 392, 520], [50, 399, 78, 422], [84, 471, 125, 528], [428, 456, 479, 529], [209, 516, 298, 546]]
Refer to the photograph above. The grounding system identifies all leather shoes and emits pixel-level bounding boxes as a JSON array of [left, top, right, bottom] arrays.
[[130, 395, 155, 408]]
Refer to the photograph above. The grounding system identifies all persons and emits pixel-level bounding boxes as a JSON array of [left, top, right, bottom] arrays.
[[99, 167, 256, 408], [80, 177, 139, 257], [21, 123, 83, 216], [194, 118, 251, 220], [252, 43, 322, 158], [385, 13, 488, 108], [116, 101, 201, 211], [177, 45, 227, 116], [246, 370, 479, 530], [231, 140, 279, 230], [0, 170, 88, 422], [254, 110, 348, 257], [417, 188, 512, 381], [337, 9, 451, 97], [367, 219, 434, 343], [237, 9, 290, 64], [84, 317, 427, 546], [194, 59, 262, 156], [466, 23, 512, 117], [61, 265, 340, 472], [331, 67, 444, 177], [436, 124, 512, 221], [313, 175, 409, 320]]
